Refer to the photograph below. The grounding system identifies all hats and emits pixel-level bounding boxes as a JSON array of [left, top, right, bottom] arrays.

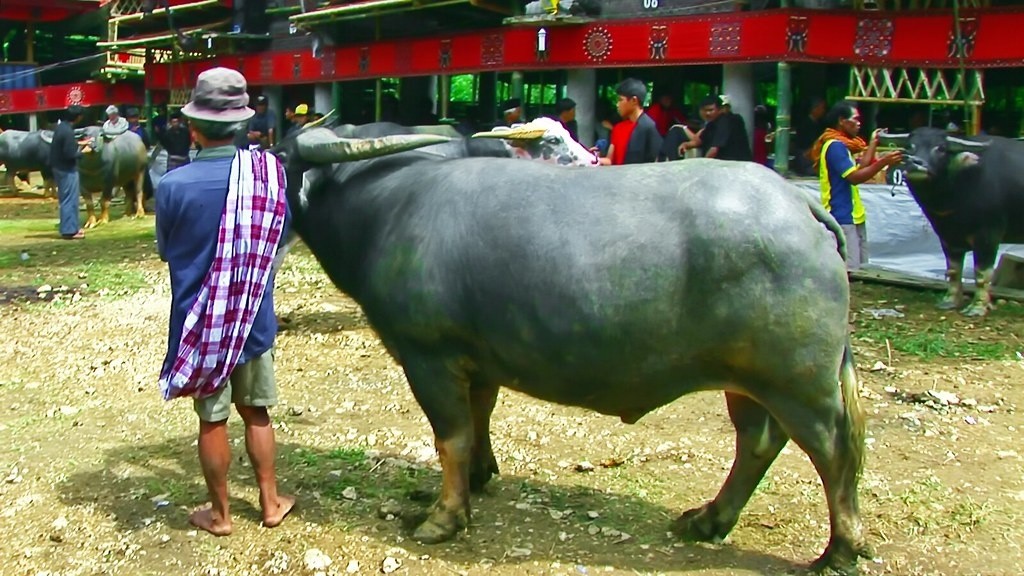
[[719, 94, 731, 105], [256, 95, 268, 104], [181, 67, 256, 124], [294, 104, 310, 115]]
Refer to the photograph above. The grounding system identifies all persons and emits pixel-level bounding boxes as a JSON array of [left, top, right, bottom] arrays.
[[102, 76, 1024, 198], [50, 105, 92, 239], [811, 101, 902, 277], [156, 66, 292, 535]]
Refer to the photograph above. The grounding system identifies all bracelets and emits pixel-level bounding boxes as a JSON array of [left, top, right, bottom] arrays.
[[269, 144, 273, 146], [682, 125, 687, 129]]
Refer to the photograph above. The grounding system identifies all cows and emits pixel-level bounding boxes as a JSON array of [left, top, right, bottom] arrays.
[[261, 109, 877, 576], [877, 122, 1023, 317], [0, 129, 58, 198], [73, 126, 148, 229]]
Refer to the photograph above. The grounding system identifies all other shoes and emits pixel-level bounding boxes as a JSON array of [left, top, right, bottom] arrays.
[[65, 231, 84, 239]]
